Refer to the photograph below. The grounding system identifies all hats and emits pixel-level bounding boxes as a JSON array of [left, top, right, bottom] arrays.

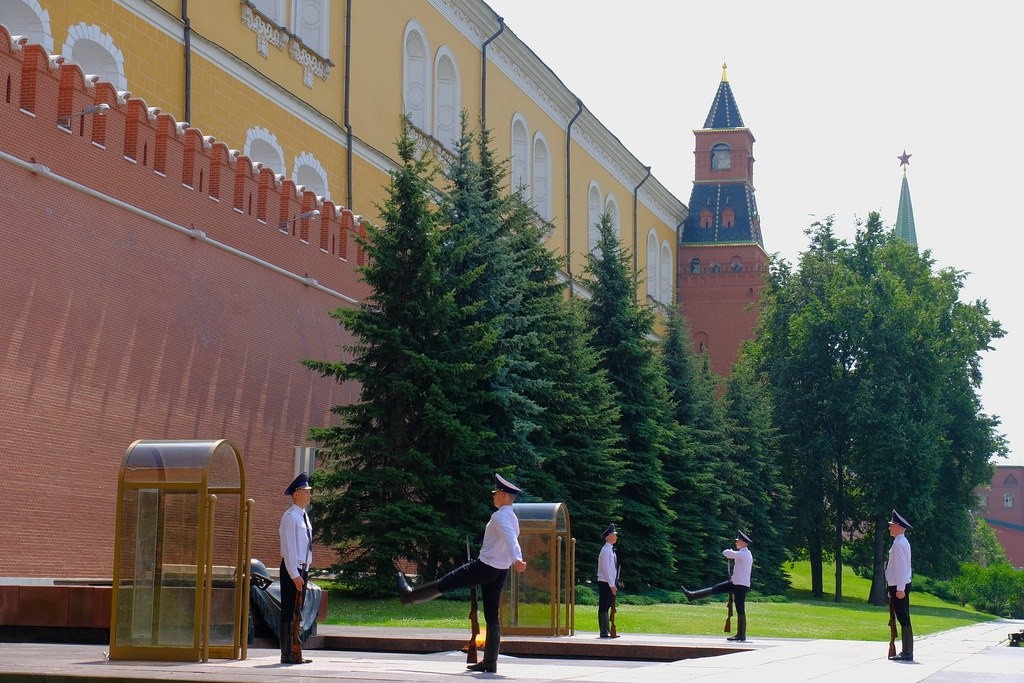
[[735, 529, 753, 544], [601, 523, 618, 538], [888, 509, 912, 529], [491, 473, 522, 495], [284, 472, 312, 495]]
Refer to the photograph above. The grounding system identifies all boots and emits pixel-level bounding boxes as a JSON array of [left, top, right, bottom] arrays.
[[681, 585, 713, 602], [727, 615, 746, 641], [889, 626, 913, 661], [598, 612, 620, 638], [279, 620, 312, 664], [467, 624, 500, 673], [397, 572, 444, 606]]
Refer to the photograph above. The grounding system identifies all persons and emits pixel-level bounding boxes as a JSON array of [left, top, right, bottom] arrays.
[[882, 510, 913, 661], [680, 529, 752, 641], [279, 472, 315, 664], [397, 473, 526, 674], [597, 522, 619, 639]]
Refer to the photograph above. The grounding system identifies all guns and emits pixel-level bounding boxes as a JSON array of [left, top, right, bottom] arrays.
[[886, 575, 897, 657], [723, 559, 734, 632], [290, 541, 312, 663], [609, 564, 621, 638], [464, 533, 481, 664]]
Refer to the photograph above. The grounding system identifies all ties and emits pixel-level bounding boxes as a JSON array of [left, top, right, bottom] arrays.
[[612, 547, 615, 562], [303, 513, 312, 551]]
